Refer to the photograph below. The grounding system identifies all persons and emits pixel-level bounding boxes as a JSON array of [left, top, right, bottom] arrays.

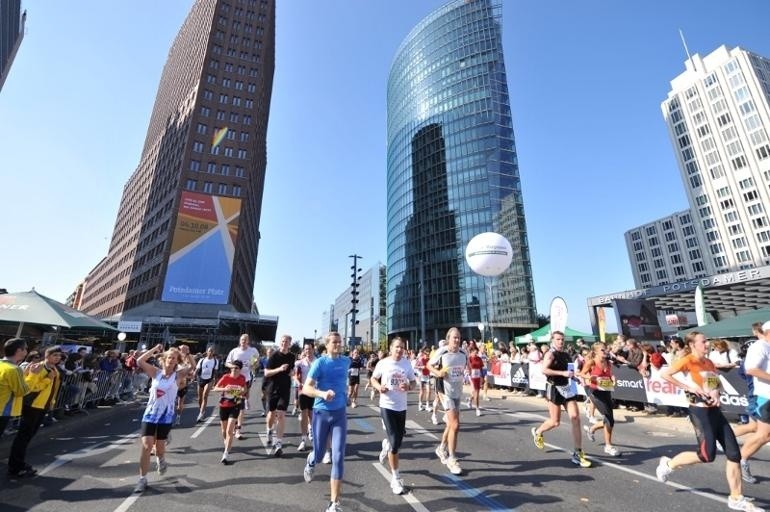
[[0, 316, 769, 512]]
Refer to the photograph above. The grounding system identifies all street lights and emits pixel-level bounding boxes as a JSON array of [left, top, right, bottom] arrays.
[[117, 333, 127, 352]]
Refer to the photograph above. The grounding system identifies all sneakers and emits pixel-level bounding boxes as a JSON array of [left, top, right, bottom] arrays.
[[175, 416, 180, 426], [741, 461, 756, 483], [158, 458, 167, 475], [350, 384, 377, 408], [150, 447, 155, 455], [322, 450, 331, 464], [657, 456, 672, 482], [292, 408, 297, 416], [245, 400, 249, 410], [728, 495, 765, 512], [267, 427, 282, 456], [297, 423, 312, 450], [165, 432, 171, 446], [8, 462, 38, 479], [304, 451, 316, 483], [571, 411, 621, 468], [419, 402, 448, 425], [378, 439, 390, 464], [531, 427, 544, 449], [435, 446, 463, 475], [391, 480, 403, 494], [196, 409, 206, 423], [466, 396, 480, 417], [325, 500, 343, 512], [232, 424, 242, 439], [134, 478, 147, 493], [221, 453, 228, 464]]
